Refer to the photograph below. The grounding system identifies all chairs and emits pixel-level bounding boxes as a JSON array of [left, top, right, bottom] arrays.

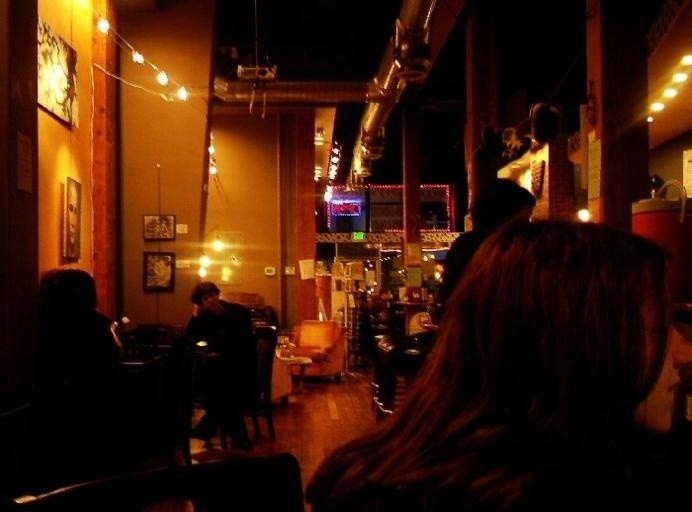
[[290, 319, 347, 383], [109, 319, 279, 469]]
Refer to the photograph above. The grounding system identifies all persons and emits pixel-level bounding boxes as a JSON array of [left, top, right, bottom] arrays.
[[35, 270, 196, 476], [67, 180, 78, 244], [182, 281, 260, 453]]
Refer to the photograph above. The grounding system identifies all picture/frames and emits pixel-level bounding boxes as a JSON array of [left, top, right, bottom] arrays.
[[143, 251, 175, 293], [142, 214, 175, 241]]
